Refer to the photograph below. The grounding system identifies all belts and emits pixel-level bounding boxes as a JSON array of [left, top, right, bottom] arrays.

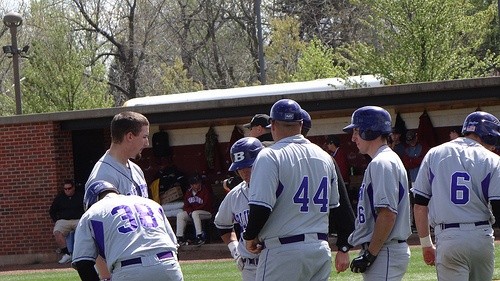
[[439, 221, 490, 229], [259, 232, 328, 249], [243, 258, 258, 265], [361, 240, 407, 250], [120, 251, 173, 267]]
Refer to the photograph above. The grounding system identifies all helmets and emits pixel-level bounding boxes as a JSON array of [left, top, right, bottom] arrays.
[[83, 181, 120, 211], [462, 112, 500, 148], [301, 109, 312, 128], [343, 106, 392, 140], [228, 137, 265, 171], [270, 99, 302, 122]]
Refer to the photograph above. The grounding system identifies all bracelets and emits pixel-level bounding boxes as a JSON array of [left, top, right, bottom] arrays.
[[420, 234, 433, 248]]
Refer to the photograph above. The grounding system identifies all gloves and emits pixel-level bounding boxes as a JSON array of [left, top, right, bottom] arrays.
[[231, 247, 244, 272], [350, 250, 376, 273]]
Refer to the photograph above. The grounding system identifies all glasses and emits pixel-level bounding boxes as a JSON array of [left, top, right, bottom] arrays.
[[64, 186, 73, 190]]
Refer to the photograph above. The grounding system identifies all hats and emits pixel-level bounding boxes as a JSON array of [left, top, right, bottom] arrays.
[[191, 176, 202, 184], [329, 138, 338, 144], [243, 114, 270, 127]]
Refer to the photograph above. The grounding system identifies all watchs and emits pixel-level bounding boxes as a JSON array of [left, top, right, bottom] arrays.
[[338, 246, 349, 253]]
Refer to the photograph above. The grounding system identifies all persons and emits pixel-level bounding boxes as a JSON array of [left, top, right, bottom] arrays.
[[176, 176, 213, 247], [342, 105, 412, 281], [214, 136, 266, 281], [222, 113, 274, 239], [71, 179, 185, 281], [302, 109, 356, 274], [326, 135, 352, 202], [389, 127, 427, 182], [49, 179, 85, 264], [449, 129, 461, 140], [243, 99, 356, 281], [83, 111, 149, 281], [408, 111, 500, 281]]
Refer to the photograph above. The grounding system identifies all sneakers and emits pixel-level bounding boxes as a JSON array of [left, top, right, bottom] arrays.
[[58, 254, 71, 264]]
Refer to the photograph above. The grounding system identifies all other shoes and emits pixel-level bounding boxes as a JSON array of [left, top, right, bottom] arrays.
[[197, 232, 206, 247], [176, 236, 185, 246]]
